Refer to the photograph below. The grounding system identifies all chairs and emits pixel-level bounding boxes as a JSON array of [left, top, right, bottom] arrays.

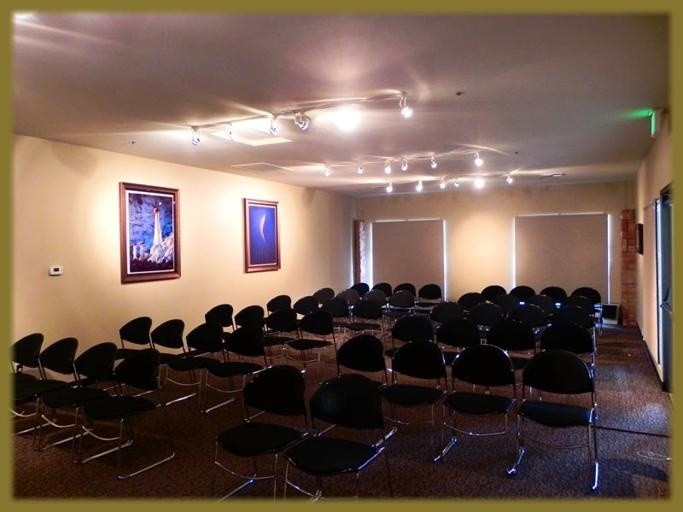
[[109, 278, 605, 451], [385, 339, 454, 462], [8, 332, 176, 483], [202, 363, 310, 498], [505, 347, 606, 493], [280, 371, 402, 500], [6, 279, 606, 502], [434, 344, 523, 476]]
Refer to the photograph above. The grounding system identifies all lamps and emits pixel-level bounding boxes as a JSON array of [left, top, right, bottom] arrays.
[[316, 142, 488, 181], [185, 88, 413, 149], [379, 171, 520, 195]]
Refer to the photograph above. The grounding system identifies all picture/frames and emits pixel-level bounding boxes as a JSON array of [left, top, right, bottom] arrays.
[[243, 195, 283, 275], [634, 221, 644, 257], [118, 180, 182, 286]]
